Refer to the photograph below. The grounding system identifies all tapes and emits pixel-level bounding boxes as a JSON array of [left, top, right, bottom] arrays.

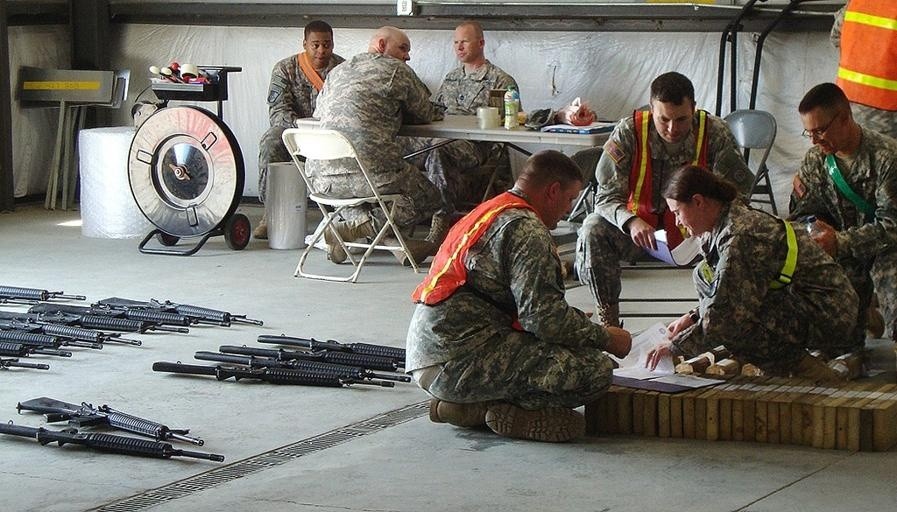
[[180, 63, 199, 81]]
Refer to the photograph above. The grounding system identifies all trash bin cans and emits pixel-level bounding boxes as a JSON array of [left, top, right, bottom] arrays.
[[266, 162, 308, 250]]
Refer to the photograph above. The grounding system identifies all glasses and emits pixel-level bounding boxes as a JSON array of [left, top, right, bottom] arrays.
[[800, 109, 843, 140]]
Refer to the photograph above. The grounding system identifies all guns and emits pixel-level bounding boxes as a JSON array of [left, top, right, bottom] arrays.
[[0, 286, 264, 370], [153, 335, 414, 387], [0, 396, 224, 462]]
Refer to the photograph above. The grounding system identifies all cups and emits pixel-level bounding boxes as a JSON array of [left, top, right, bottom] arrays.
[[475, 106, 499, 128]]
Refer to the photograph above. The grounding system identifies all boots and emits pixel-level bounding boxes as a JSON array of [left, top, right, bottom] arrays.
[[793, 356, 846, 386], [484, 403, 585, 442], [423, 215, 452, 257], [864, 292, 885, 340], [253, 204, 267, 237], [430, 398, 487, 428], [380, 231, 434, 267], [324, 214, 373, 264]]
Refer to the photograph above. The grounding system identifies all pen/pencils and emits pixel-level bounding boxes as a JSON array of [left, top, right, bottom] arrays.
[[620, 320, 623, 329]]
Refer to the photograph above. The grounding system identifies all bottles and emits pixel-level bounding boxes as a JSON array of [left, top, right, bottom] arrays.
[[504, 84, 520, 129], [807, 214, 823, 236]]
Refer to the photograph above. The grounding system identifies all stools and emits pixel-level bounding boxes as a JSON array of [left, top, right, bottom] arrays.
[[602, 246, 709, 331], [456, 143, 515, 211]]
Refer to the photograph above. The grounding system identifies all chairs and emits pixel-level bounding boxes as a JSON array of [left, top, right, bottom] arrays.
[[721, 107, 777, 215], [534, 144, 602, 288], [280, 125, 423, 280]]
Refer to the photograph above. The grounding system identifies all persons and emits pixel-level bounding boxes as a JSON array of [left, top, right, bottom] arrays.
[[575, 72, 755, 328], [646, 165, 861, 376], [780, 82, 897, 344], [405, 23, 523, 256], [252, 21, 346, 240], [404, 149, 631, 442], [304, 25, 441, 265], [830, 0, 897, 139]]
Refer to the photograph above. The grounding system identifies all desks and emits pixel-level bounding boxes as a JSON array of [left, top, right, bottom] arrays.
[[293, 113, 618, 220]]
